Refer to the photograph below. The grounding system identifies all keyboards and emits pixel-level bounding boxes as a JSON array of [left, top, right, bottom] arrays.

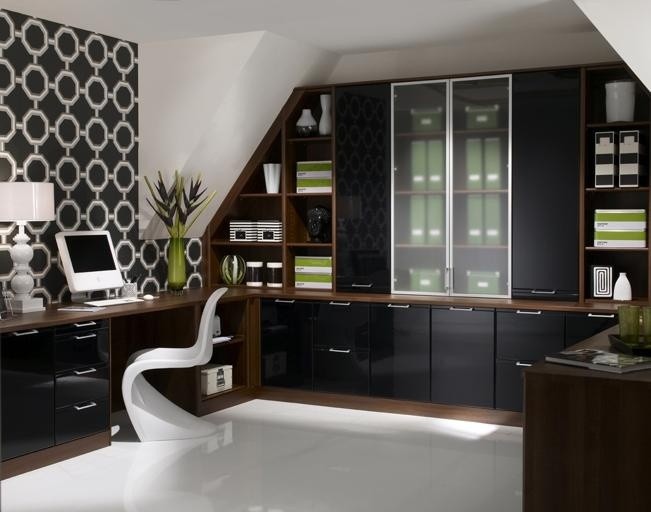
[[85, 297, 144, 306]]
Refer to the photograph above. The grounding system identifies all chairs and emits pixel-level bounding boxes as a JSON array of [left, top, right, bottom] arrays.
[[121, 287, 230, 442]]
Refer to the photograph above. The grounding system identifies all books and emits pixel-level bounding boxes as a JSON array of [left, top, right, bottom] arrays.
[[543, 347, 651, 374]]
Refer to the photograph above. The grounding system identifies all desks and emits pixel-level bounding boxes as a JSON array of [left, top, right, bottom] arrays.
[[0, 287, 261, 335]]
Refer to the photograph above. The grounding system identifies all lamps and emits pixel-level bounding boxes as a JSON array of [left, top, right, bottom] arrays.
[[0, 182, 56, 315]]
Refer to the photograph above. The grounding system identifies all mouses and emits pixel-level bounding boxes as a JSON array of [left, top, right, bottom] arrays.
[[144, 293, 153, 301]]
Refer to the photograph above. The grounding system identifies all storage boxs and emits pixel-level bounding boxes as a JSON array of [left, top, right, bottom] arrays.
[[201, 364, 233, 396]]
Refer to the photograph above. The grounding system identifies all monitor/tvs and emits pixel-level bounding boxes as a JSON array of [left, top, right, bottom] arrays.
[[56, 229, 126, 302]]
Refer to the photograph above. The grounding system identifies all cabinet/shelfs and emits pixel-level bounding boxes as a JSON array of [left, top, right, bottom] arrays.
[[147, 297, 261, 417], [1, 319, 110, 482]]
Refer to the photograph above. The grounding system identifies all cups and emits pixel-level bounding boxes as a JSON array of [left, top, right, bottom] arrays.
[[262, 163, 282, 194], [246, 260, 264, 287], [267, 262, 281, 288]]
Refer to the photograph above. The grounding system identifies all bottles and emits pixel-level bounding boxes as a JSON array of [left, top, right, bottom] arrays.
[[613, 272, 632, 300]]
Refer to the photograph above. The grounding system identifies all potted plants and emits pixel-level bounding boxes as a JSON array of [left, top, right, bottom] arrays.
[[141, 170, 217, 295]]
